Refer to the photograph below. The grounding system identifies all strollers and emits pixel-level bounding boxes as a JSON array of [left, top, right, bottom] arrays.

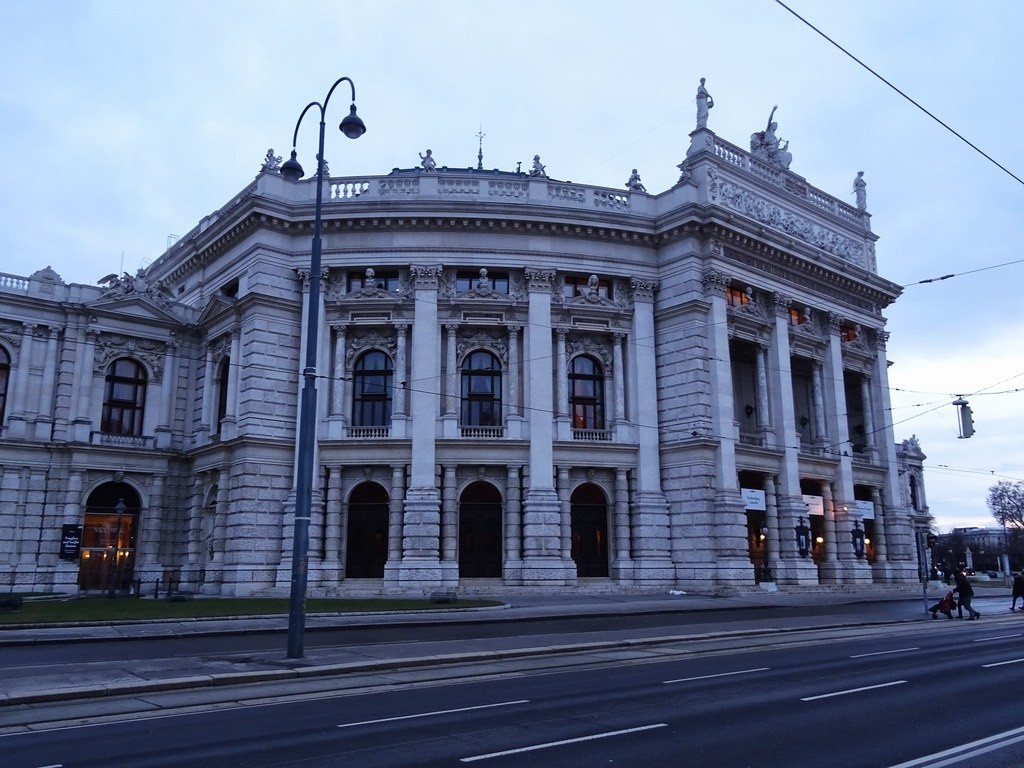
[[929, 589, 956, 620]]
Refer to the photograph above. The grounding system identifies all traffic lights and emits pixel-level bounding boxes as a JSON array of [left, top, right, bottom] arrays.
[[927, 531, 937, 547]]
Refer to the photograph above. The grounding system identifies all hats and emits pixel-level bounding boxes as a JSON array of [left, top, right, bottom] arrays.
[[946, 591, 953, 599]]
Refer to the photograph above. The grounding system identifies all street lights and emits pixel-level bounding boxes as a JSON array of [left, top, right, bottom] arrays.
[[107, 498, 127, 599], [279, 74, 366, 659]]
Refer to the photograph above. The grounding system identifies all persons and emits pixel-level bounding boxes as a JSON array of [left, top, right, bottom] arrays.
[[95, 268, 177, 314], [419, 149, 436, 172], [929, 562, 1024, 620], [263, 148, 282, 169], [314, 154, 329, 175], [696, 78, 713, 127], [364, 268, 376, 288], [625, 169, 648, 192], [853, 325, 860, 337], [588, 275, 599, 293], [804, 308, 810, 321], [762, 106, 793, 169], [477, 269, 488, 288], [853, 171, 867, 212], [745, 288, 753, 300], [531, 155, 547, 178]]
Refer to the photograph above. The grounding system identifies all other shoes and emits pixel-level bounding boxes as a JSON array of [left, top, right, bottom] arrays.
[[1019, 607, 1024, 610], [956, 616, 963, 618], [965, 617, 975, 620], [1009, 608, 1014, 611], [976, 612, 980, 619]]
